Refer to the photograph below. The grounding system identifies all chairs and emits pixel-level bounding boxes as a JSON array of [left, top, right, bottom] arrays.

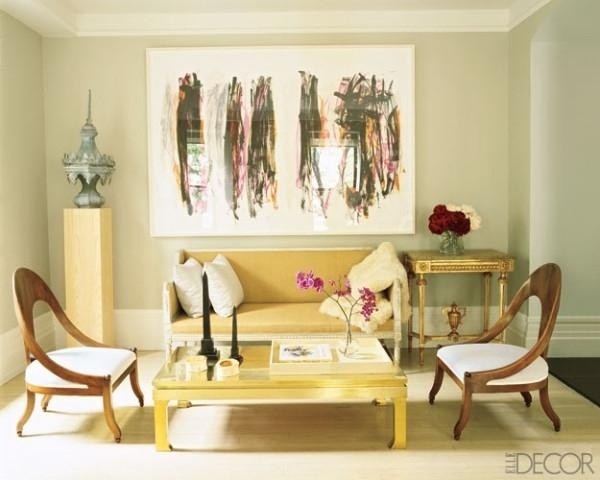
[[12, 267, 144, 443], [429, 263, 561, 441]]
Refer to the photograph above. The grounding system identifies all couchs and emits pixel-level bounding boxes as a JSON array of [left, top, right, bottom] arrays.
[[162, 246, 402, 366]]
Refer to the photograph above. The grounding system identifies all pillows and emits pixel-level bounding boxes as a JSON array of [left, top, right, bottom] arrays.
[[202, 253, 244, 318], [174, 257, 210, 318]]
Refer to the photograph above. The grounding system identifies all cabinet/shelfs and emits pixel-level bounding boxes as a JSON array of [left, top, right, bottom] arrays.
[[63, 208, 115, 348]]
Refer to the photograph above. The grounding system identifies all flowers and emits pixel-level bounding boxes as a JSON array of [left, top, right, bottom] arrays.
[[295, 269, 379, 356], [428, 203, 482, 237]]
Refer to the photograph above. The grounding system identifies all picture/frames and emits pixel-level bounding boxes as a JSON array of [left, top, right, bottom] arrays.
[[146, 45, 416, 237]]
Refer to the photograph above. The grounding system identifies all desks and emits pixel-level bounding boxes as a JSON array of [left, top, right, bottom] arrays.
[[403, 249, 516, 367]]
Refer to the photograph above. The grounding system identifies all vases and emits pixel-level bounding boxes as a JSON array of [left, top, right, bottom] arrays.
[[336, 338, 361, 363], [439, 231, 464, 255]]
